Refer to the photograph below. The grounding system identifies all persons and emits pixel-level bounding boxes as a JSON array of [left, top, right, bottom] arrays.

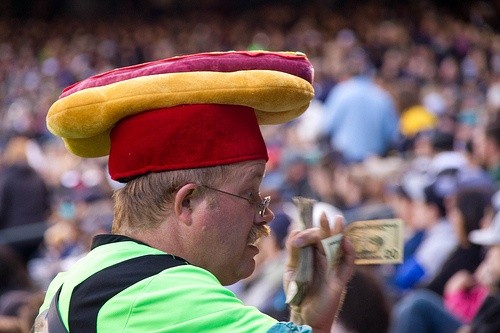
[[222, 14, 499, 333], [33, 132, 355, 333], [2, 17, 222, 318]]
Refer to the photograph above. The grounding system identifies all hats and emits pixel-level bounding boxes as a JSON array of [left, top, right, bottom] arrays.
[[44, 48, 313, 183], [318, 148, 500, 270]]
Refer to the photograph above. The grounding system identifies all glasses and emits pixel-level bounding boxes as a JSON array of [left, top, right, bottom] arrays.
[[174, 181, 270, 216]]
